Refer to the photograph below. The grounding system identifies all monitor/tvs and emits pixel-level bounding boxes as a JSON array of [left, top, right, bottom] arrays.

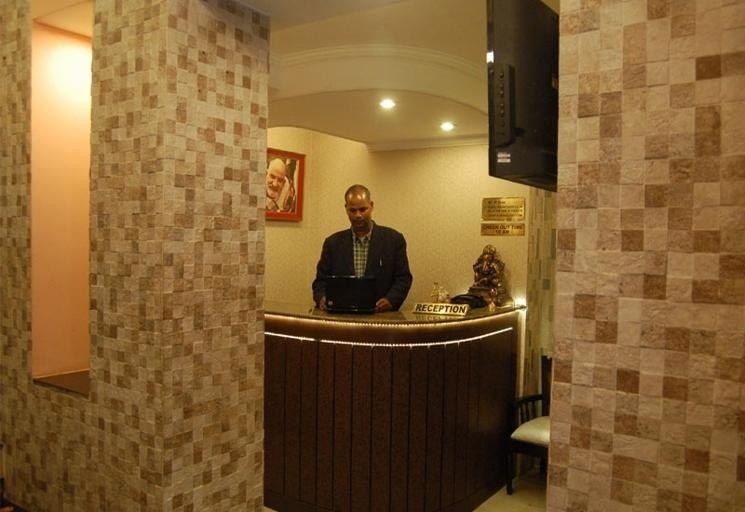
[[486, 0, 560, 192]]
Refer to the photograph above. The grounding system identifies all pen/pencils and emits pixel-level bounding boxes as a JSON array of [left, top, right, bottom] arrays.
[[380, 259, 382, 269]]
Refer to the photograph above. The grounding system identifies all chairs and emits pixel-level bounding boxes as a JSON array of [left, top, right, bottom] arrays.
[[501, 355, 552, 495]]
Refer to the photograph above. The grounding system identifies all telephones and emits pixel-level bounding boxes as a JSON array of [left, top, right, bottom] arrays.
[[450, 295, 486, 308]]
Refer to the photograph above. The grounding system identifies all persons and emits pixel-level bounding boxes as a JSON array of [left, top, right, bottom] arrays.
[[265, 157, 294, 213], [312, 184, 413, 313]]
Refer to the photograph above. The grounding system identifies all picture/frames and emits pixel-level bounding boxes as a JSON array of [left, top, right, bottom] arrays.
[[265, 147, 305, 222]]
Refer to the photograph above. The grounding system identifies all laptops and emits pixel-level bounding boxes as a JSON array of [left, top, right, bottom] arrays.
[[324, 273, 380, 315]]
